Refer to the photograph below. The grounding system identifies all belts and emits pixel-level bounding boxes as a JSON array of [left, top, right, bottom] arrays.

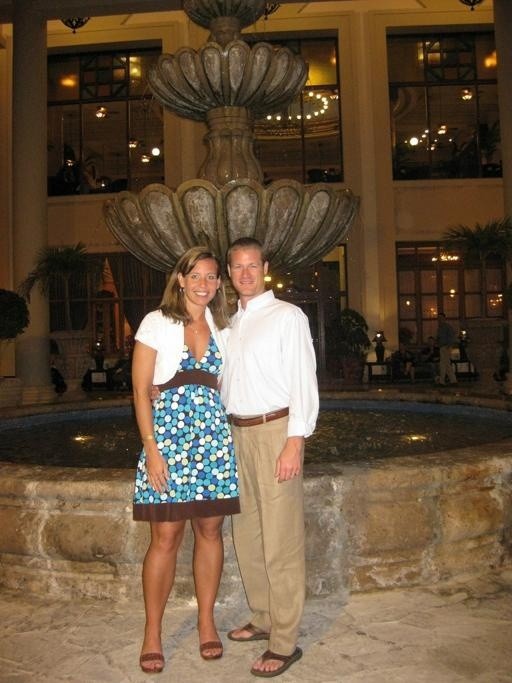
[[228, 406, 288, 426]]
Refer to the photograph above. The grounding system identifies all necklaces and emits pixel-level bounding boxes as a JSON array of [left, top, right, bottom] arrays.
[[187, 322, 202, 335]]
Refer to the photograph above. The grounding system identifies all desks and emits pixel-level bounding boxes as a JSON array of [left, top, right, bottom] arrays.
[[364, 361, 390, 384], [450, 357, 472, 381], [85, 365, 108, 392]]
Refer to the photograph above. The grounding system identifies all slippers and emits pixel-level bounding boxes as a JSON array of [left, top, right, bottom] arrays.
[[227, 622, 270, 641], [250, 646, 302, 676], [200, 640, 223, 659], [140, 652, 165, 672]]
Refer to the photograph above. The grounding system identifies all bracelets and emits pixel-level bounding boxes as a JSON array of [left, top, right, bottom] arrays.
[[140, 435, 155, 440]]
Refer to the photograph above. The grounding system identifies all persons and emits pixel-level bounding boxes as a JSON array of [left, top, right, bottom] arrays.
[[435, 311, 461, 387], [394, 340, 418, 387], [420, 337, 440, 364], [130, 247, 241, 676], [62, 143, 75, 167], [150, 236, 320, 680]]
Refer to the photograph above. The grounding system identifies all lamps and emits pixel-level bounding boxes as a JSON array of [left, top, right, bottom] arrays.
[[371, 329, 387, 361]]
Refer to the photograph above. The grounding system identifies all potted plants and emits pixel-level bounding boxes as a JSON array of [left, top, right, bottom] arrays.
[[332, 306, 371, 384], [440, 212, 511, 371], [14, 240, 108, 380]]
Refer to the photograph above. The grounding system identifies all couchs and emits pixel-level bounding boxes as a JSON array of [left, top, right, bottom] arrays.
[[386, 351, 431, 383]]
